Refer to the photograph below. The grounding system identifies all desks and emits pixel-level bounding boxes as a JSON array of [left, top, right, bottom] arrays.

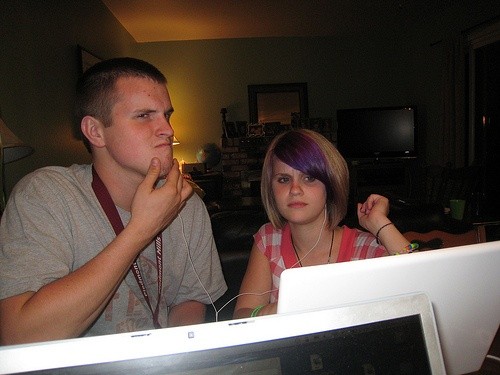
[[348, 164, 500, 243]]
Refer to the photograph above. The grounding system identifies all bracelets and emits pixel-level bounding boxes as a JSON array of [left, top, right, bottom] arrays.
[[375, 223, 394, 246], [251, 304, 264, 317]]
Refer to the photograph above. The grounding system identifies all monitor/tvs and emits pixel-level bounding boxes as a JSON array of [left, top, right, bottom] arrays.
[[336, 102, 420, 159], [0, 295, 447, 374], [278, 242, 500, 375]]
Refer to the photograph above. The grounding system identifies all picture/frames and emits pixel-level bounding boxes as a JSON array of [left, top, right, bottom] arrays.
[[75, 46, 105, 77]]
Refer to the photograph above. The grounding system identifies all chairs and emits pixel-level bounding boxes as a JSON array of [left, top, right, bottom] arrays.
[[427, 167, 464, 203]]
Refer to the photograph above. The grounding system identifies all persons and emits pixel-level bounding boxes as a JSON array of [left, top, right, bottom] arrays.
[[0, 57, 227, 346], [234, 129, 419, 318]]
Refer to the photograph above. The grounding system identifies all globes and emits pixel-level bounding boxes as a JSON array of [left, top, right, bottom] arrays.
[[197, 143, 221, 173]]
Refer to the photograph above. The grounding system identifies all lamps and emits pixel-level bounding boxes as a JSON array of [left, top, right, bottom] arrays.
[[0, 118, 35, 215]]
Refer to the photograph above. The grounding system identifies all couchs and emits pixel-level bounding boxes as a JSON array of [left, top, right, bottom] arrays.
[[206, 211, 268, 324]]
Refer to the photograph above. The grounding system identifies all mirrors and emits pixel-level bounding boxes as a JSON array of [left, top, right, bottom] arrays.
[[246, 81, 310, 126]]
